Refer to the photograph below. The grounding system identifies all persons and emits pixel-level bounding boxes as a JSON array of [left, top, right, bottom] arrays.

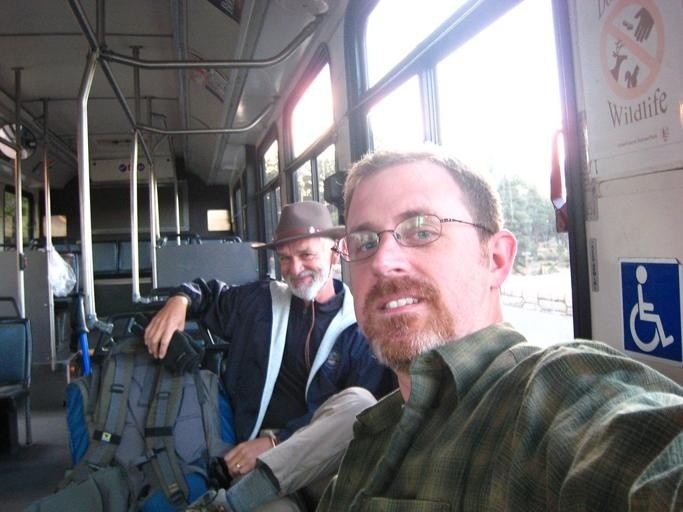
[[312, 139, 683, 512], [142, 200, 395, 512]]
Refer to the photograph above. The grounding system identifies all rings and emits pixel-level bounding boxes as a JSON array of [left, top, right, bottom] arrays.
[[236, 463, 240, 468]]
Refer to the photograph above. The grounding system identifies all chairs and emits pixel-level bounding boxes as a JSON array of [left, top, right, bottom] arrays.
[[0, 231, 275, 453]]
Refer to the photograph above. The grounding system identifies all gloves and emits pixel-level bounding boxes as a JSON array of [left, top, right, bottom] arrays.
[[131, 312, 203, 376]]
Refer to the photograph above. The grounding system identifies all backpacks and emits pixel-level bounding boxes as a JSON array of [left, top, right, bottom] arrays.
[[65, 332, 237, 512]]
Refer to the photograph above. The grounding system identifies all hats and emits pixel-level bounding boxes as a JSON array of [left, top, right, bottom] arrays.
[[251, 200, 346, 248]]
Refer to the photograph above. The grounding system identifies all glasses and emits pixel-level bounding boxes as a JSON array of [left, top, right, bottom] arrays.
[[331, 213, 492, 261]]
